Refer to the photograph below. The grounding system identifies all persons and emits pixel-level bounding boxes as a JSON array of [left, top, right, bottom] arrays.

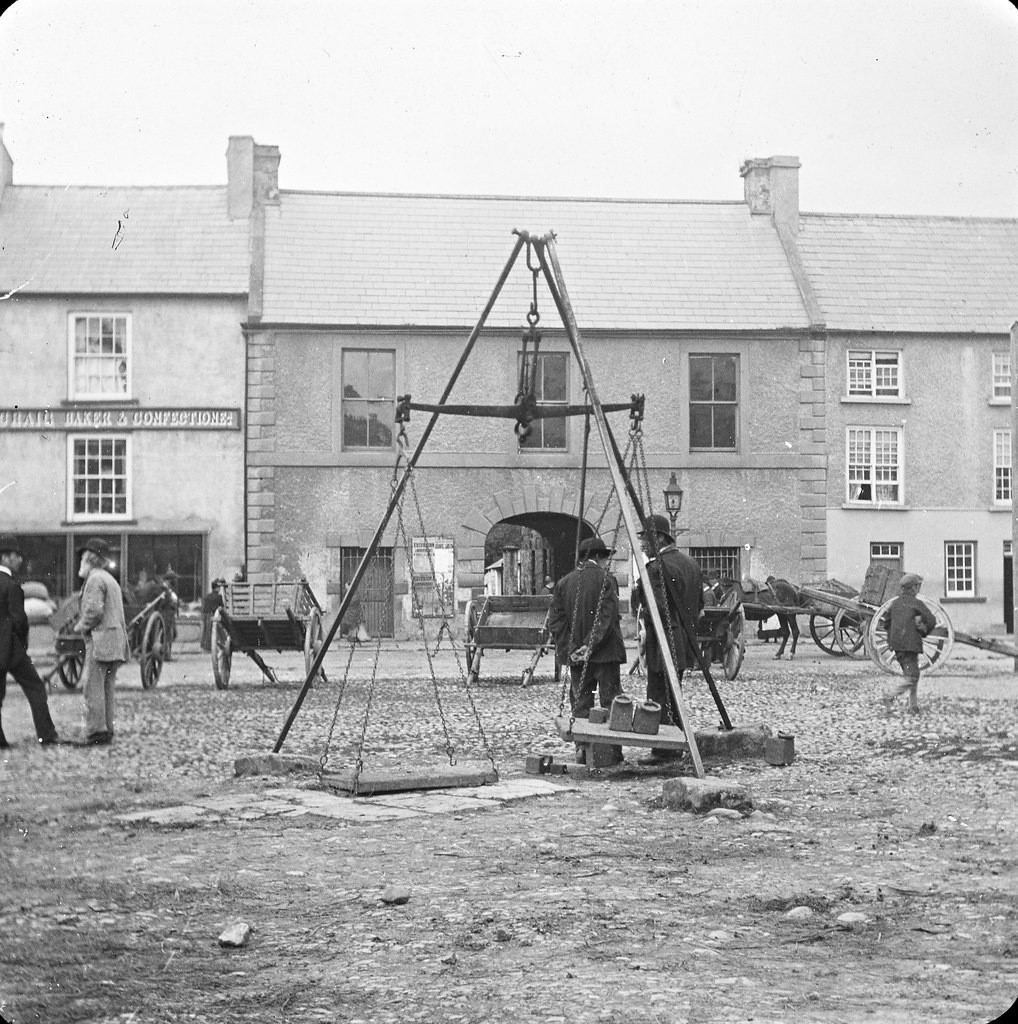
[[0, 533, 71, 750], [159, 571, 180, 662], [541, 576, 555, 595], [708, 570, 724, 602], [882, 573, 936, 711], [340, 581, 365, 642], [701, 574, 717, 607], [637, 514, 706, 766], [70, 538, 131, 747], [200, 580, 224, 651], [548, 537, 627, 764], [718, 578, 736, 608], [145, 567, 167, 602]]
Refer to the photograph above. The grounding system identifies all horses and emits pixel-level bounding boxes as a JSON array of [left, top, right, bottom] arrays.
[[717, 576, 800, 660]]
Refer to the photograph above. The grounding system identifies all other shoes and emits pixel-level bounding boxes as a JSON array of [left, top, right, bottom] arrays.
[[637, 754, 680, 766], [40, 733, 71, 745], [883, 693, 894, 709], [70, 733, 113, 747], [907, 706, 919, 715]]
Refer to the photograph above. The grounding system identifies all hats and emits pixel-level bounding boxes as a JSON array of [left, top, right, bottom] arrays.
[[899, 573, 922, 589], [636, 515, 676, 542], [0, 534, 20, 551], [81, 538, 109, 561], [570, 537, 616, 559]]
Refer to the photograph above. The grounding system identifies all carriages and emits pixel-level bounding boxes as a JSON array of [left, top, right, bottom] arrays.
[[629, 563, 891, 676]]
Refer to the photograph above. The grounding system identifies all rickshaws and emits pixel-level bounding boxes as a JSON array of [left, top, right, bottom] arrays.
[[210, 576, 325, 691], [464, 595, 562, 687], [39, 583, 177, 699]]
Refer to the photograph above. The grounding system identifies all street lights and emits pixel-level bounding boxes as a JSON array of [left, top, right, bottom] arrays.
[[664, 472, 689, 544]]
